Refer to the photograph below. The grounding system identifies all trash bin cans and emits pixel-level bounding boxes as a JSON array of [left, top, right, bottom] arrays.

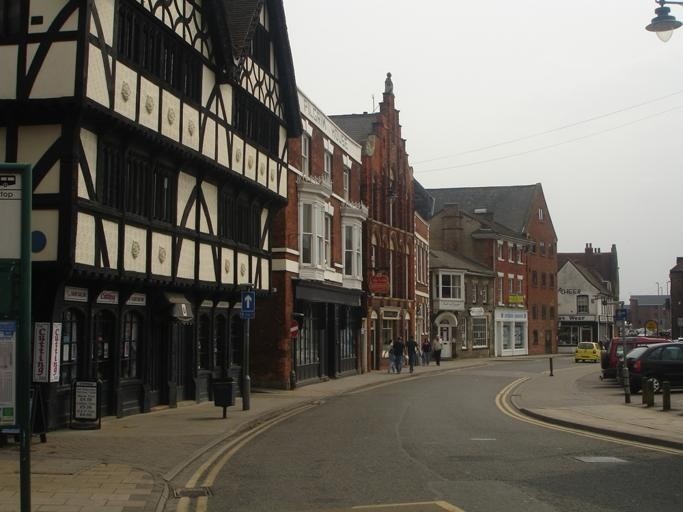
[[441, 341, 451, 358]]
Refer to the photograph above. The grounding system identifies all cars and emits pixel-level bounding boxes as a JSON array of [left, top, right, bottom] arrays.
[[574, 335, 682, 394]]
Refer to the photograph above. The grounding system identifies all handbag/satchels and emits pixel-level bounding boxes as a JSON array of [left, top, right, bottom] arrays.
[[381, 350, 389, 359]]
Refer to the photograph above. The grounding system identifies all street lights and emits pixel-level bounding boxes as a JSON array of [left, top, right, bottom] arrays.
[[238, 282, 255, 411]]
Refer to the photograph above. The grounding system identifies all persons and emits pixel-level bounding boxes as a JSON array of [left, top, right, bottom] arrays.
[[384, 335, 445, 374]]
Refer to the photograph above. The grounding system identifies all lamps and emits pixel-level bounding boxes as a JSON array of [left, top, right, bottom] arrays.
[[644, 0, 683, 44]]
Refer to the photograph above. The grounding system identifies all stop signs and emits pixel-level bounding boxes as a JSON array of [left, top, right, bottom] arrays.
[[289, 319, 299, 338]]
